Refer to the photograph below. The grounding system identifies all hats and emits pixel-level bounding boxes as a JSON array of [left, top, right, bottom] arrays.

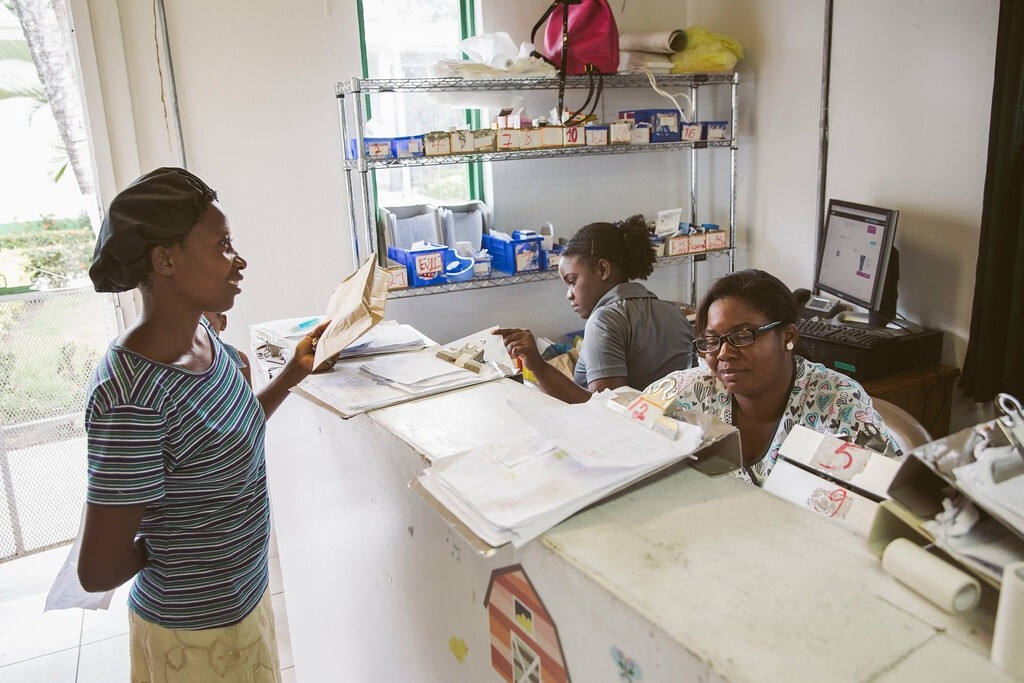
[[89, 168, 216, 292]]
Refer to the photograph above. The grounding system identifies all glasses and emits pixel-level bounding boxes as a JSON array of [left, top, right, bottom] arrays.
[[691, 319, 780, 353]]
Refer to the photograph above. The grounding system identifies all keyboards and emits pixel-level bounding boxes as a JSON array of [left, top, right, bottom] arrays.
[[793, 318, 888, 348]]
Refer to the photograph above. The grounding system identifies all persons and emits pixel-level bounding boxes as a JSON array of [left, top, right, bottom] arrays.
[[491, 214, 699, 405], [639, 269, 904, 490], [77, 169, 339, 683]]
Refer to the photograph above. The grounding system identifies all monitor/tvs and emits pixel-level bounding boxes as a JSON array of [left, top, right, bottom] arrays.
[[813, 198, 924, 337]]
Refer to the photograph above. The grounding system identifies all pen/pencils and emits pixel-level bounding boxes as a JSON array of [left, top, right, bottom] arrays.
[[285, 316, 320, 333]]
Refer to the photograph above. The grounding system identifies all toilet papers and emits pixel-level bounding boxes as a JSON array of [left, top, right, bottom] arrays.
[[877, 537, 981, 632], [989, 559, 1022, 680]]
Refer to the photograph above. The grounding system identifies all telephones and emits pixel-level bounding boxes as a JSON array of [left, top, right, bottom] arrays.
[[788, 287, 842, 329]]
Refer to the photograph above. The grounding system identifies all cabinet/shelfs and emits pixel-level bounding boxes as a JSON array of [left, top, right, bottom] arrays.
[[334, 69, 739, 307], [862, 362, 961, 439]]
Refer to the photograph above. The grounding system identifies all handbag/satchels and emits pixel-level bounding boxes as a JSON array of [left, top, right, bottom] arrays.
[[530, 0, 620, 128]]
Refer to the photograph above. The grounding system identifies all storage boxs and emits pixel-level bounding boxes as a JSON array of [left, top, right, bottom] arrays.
[[541, 126, 563, 149], [619, 108, 685, 142], [482, 230, 544, 274], [496, 127, 519, 152], [393, 135, 424, 158], [585, 125, 610, 146], [681, 121, 706, 140], [473, 251, 493, 276], [697, 121, 728, 140], [424, 132, 450, 157], [449, 130, 475, 155], [563, 126, 585, 148], [685, 228, 727, 255], [541, 242, 565, 271], [377, 256, 409, 290], [388, 241, 449, 288], [663, 234, 688, 257], [350, 137, 396, 160], [600, 120, 632, 145], [491, 107, 599, 130], [519, 126, 543, 151]]
